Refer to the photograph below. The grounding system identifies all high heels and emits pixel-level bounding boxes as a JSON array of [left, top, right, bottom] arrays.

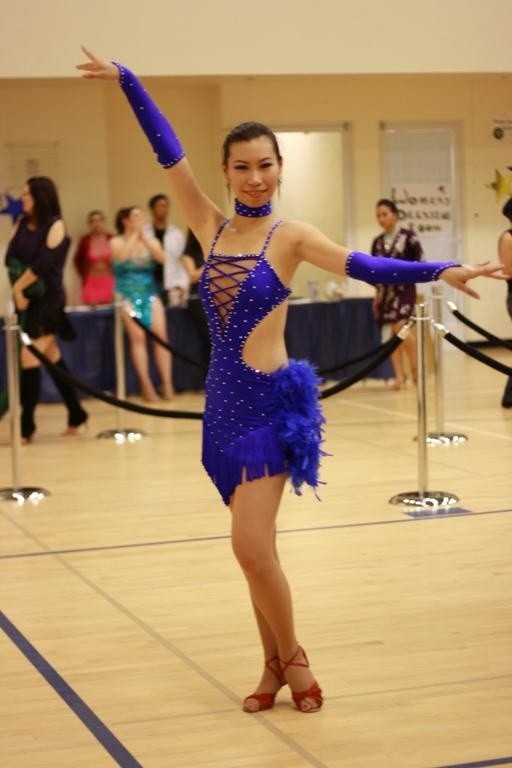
[[392, 373, 417, 388], [61, 417, 89, 437]]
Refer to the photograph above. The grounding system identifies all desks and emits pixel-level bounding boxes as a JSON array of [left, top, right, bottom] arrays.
[[0, 291, 429, 414]]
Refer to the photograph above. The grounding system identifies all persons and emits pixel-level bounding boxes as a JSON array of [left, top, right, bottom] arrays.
[[71, 38, 511, 713], [106, 203, 175, 403], [3, 172, 90, 445], [366, 197, 425, 393], [141, 191, 206, 304], [494, 198, 512, 408], [72, 207, 116, 307]]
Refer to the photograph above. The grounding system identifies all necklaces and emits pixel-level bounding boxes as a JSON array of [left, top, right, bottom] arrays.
[[234, 197, 271, 218]]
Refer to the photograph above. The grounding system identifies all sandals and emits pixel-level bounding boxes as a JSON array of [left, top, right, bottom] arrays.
[[244, 647, 323, 713]]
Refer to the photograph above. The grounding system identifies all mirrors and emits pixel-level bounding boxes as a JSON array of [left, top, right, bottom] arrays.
[[226, 122, 354, 303]]
